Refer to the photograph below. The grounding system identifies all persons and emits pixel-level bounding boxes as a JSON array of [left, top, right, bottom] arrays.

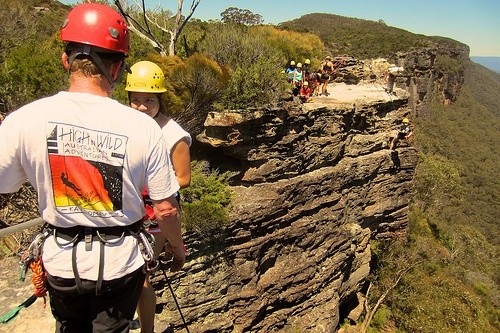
[[0, 3, 193, 333], [280, 56, 334, 102]]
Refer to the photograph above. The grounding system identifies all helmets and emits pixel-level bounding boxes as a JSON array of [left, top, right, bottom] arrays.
[[297, 62, 302, 67], [290, 60, 296, 65], [59, 2, 132, 59], [125, 60, 168, 92], [305, 58, 311, 64], [303, 81, 308, 85]]
